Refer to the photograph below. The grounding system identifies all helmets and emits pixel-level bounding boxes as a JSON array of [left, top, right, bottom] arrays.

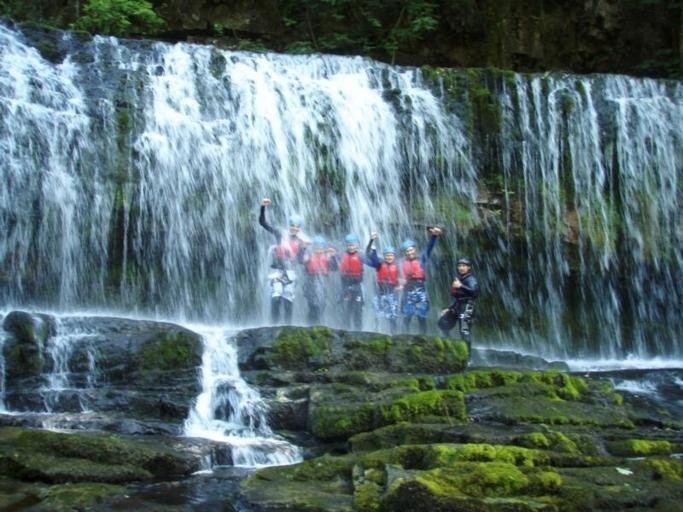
[[312, 236, 326, 246], [383, 246, 395, 254], [400, 239, 417, 251], [456, 256, 472, 266], [345, 233, 359, 243], [289, 217, 303, 227]]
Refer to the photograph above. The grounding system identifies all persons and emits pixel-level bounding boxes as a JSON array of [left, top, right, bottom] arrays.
[[298, 236, 339, 325], [337, 234, 374, 331], [366, 232, 400, 335], [401, 227, 442, 336], [259, 198, 306, 325], [437, 256, 480, 367]]
